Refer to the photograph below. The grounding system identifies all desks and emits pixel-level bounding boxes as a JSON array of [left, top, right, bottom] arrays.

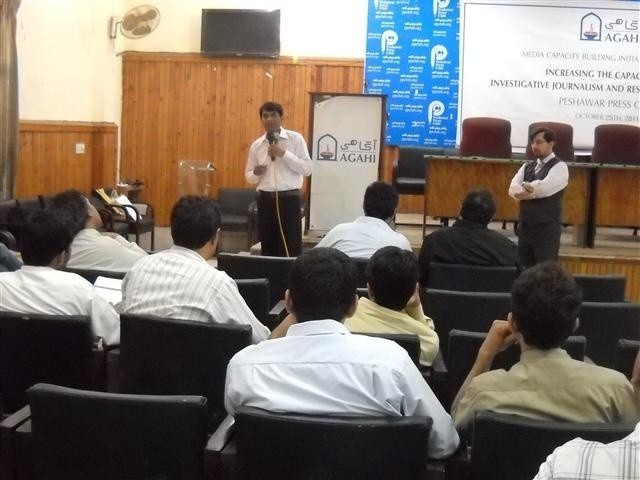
[[422, 152, 594, 249], [589, 163, 640, 250]]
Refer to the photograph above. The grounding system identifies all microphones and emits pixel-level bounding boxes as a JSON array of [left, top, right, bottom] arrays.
[[266, 132, 276, 161]]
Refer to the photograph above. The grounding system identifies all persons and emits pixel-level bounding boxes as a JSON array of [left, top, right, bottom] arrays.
[[535, 344, 640, 480], [123, 195, 296, 346], [510, 128, 569, 267], [345, 247, 440, 367], [225, 248, 460, 462], [0, 210, 121, 349], [245, 102, 313, 258], [418, 189, 519, 288], [317, 181, 413, 259], [48, 189, 149, 271], [0, 244, 22, 272], [453, 260, 639, 433]]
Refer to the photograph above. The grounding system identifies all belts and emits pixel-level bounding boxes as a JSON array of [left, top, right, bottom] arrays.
[[257, 190, 299, 197]]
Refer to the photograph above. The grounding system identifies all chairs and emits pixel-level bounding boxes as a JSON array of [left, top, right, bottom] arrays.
[[447, 408, 634, 477], [459, 116, 513, 229], [216, 251, 372, 331], [220, 405, 448, 477], [526, 121, 577, 229], [351, 331, 432, 387], [421, 261, 640, 412], [212, 188, 305, 255], [0, 194, 57, 251], [66, 266, 127, 285], [590, 123, 640, 236], [393, 144, 449, 228], [0, 310, 253, 477], [85, 187, 155, 251]]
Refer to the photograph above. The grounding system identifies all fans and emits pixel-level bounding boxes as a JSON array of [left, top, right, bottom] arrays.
[[109, 3, 161, 40]]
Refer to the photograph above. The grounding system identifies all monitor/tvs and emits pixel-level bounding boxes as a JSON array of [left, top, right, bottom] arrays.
[[201, 8, 280, 59]]
